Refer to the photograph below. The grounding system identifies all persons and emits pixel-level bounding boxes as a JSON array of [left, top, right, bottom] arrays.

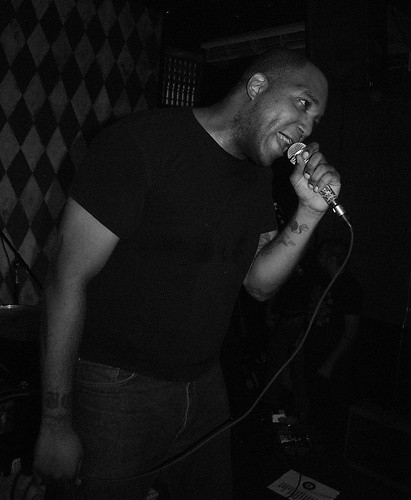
[[33, 47, 341, 500]]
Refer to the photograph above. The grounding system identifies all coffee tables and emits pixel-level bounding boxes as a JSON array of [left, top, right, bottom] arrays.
[[233, 455, 410, 499]]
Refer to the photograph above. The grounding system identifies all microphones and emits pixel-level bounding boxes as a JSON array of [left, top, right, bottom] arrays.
[[287, 141, 353, 229]]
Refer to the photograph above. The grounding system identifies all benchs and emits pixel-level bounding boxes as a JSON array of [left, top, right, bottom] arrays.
[[371, 318, 410, 482]]
